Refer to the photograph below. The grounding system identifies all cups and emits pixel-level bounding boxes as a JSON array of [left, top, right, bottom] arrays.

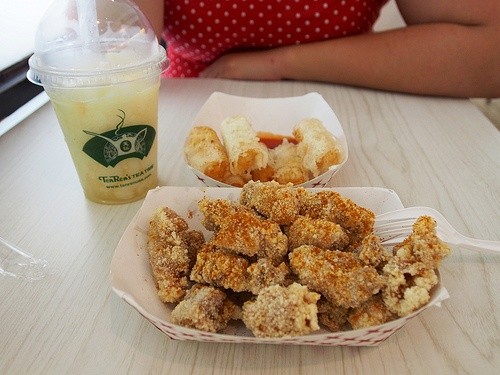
[[25, 0, 171, 207]]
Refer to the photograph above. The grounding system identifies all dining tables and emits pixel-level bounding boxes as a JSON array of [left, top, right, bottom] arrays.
[[1, 79, 500, 374]]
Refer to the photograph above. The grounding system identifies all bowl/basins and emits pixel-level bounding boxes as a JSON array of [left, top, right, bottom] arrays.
[[184, 91, 348, 190], [107, 183, 451, 346]]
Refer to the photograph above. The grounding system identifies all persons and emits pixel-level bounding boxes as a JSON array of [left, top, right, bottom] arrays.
[[68, 1, 500, 99]]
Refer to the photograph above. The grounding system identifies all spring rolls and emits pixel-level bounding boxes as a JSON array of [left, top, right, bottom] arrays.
[[184, 113, 342, 187]]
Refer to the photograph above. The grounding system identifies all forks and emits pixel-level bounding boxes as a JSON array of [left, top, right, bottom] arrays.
[[372, 206, 500, 256]]
